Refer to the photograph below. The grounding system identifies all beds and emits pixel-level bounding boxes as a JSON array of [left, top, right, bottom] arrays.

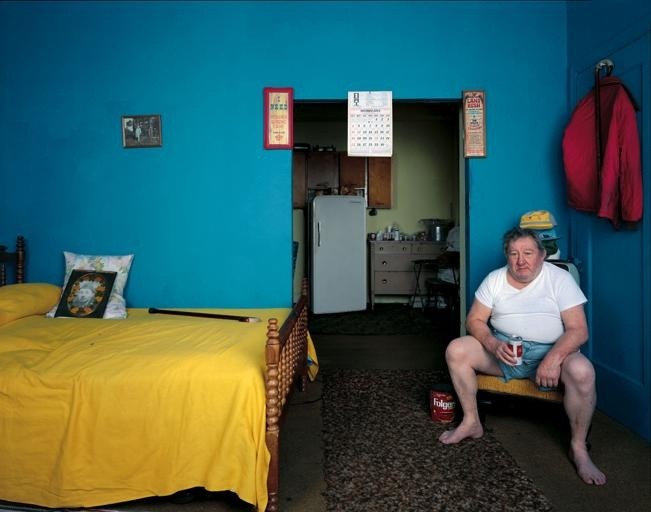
[[0, 231, 319, 510]]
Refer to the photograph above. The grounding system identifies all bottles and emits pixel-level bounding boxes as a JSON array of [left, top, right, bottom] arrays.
[[370, 225, 427, 242]]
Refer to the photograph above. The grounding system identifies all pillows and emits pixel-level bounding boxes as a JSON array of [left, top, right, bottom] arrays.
[[0, 280, 60, 330], [43, 250, 136, 322]]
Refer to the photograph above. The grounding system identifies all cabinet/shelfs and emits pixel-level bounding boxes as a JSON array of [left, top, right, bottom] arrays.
[[293, 142, 309, 209], [366, 155, 394, 210], [339, 150, 368, 210], [369, 239, 448, 309], [306, 144, 339, 201]]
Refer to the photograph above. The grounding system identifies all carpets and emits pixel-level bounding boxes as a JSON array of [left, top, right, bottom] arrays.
[[316, 364, 554, 512]]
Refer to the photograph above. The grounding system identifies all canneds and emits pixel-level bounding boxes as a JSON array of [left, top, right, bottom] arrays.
[[508, 335, 523, 366]]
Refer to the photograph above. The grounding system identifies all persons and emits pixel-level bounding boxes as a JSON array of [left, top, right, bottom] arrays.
[[134, 125, 142, 144], [438, 225, 607, 485], [74, 286, 95, 308]]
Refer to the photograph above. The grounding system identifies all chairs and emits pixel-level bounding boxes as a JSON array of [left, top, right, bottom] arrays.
[[439, 250, 460, 312]]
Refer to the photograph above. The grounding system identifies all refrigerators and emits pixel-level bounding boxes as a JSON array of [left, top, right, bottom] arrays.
[[312, 194, 367, 316]]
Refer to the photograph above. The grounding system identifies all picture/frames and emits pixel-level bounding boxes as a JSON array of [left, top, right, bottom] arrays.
[[120, 114, 162, 148]]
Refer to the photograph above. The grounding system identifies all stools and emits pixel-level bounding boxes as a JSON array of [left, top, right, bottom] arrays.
[[474, 371, 592, 454], [424, 278, 448, 312]]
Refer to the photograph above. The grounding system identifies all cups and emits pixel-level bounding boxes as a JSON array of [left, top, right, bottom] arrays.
[[430, 387, 458, 425]]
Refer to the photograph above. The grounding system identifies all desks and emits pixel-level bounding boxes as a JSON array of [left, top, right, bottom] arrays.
[[405, 258, 439, 310]]
